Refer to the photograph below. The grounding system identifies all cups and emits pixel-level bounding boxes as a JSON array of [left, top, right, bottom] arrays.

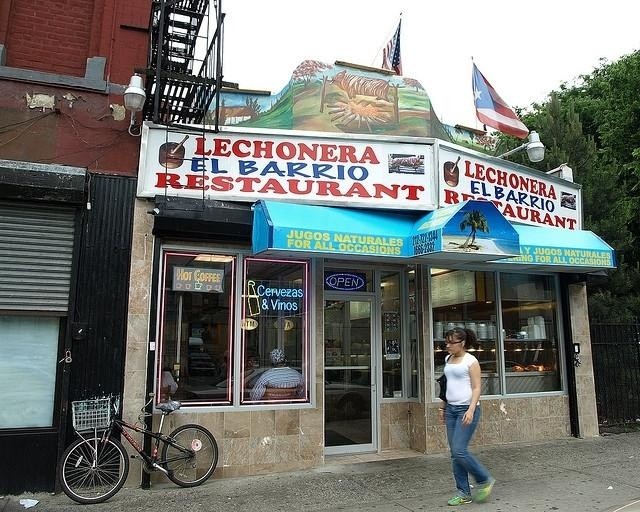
[[159, 142, 186, 169], [444, 160, 460, 187]]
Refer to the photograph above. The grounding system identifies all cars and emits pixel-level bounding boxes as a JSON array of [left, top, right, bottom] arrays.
[[479, 360, 525, 372], [181, 367, 370, 419], [187, 336, 218, 384]]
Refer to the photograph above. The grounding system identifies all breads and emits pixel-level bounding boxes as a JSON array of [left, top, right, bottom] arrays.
[[511, 364, 549, 373]]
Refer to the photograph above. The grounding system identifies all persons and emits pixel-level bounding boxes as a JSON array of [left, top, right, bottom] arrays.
[[162, 371, 178, 401], [437, 326, 496, 505], [251, 348, 309, 403]]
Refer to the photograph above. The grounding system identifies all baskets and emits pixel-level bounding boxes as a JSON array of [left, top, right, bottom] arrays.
[[71, 399, 112, 431]]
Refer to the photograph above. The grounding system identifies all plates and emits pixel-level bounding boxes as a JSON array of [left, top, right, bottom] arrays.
[[433, 315, 547, 342]]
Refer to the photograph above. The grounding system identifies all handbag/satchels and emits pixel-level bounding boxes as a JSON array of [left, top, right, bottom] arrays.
[[437, 374, 447, 402]]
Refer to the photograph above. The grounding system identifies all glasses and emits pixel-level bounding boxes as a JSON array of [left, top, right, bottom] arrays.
[[445, 341, 461, 345]]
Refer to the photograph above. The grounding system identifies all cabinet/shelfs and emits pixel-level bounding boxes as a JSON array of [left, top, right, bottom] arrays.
[[434, 339, 558, 398]]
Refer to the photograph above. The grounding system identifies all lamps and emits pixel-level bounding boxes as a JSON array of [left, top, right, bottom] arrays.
[[497, 130, 545, 163], [123, 73, 147, 136]]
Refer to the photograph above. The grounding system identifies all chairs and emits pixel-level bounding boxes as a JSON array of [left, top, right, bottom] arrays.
[[261, 387, 300, 403]]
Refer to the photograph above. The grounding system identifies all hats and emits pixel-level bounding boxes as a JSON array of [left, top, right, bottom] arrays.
[[270, 349, 285, 364]]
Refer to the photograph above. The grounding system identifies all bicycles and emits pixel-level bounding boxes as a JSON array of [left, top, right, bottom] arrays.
[[56, 391, 220, 506]]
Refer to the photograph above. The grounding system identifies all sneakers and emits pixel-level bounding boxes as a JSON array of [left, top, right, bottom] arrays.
[[477, 480, 495, 502], [448, 496, 472, 506]]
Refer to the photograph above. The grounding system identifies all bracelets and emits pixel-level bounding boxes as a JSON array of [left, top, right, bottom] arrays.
[[438, 408, 446, 411]]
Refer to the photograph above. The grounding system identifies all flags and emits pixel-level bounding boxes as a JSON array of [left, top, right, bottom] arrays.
[[472, 61, 530, 139], [382, 18, 402, 75]]
